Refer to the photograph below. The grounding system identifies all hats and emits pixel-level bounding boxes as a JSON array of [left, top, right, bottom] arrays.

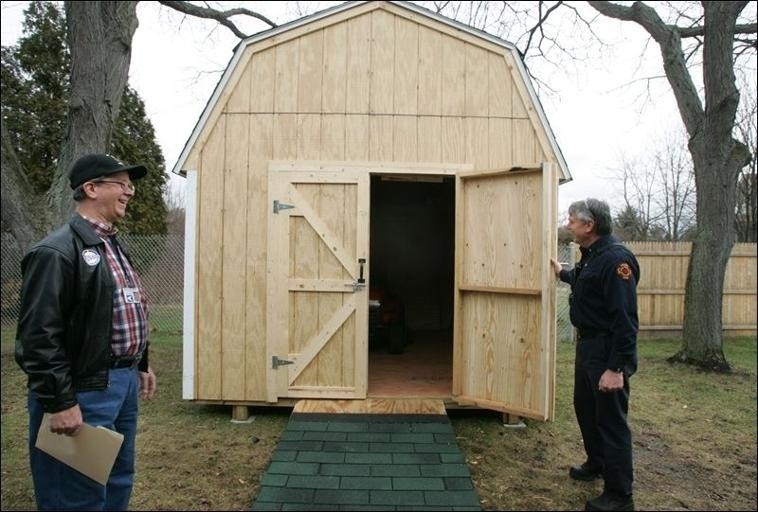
[[68, 153, 148, 190]]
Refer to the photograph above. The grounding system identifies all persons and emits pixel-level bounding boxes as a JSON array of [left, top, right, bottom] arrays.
[[550, 197, 640, 511], [14, 155, 156, 511]]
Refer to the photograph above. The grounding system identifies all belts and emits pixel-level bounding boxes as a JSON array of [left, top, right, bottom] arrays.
[[109, 355, 142, 370]]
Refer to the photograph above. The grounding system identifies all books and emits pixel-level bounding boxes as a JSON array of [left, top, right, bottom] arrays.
[[34, 413, 125, 487]]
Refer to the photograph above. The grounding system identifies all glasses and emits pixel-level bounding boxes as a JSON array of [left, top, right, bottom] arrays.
[[93, 180, 136, 193]]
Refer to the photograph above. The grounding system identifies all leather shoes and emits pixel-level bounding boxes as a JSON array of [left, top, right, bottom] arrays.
[[585, 492, 635, 512], [569, 457, 602, 481]]
